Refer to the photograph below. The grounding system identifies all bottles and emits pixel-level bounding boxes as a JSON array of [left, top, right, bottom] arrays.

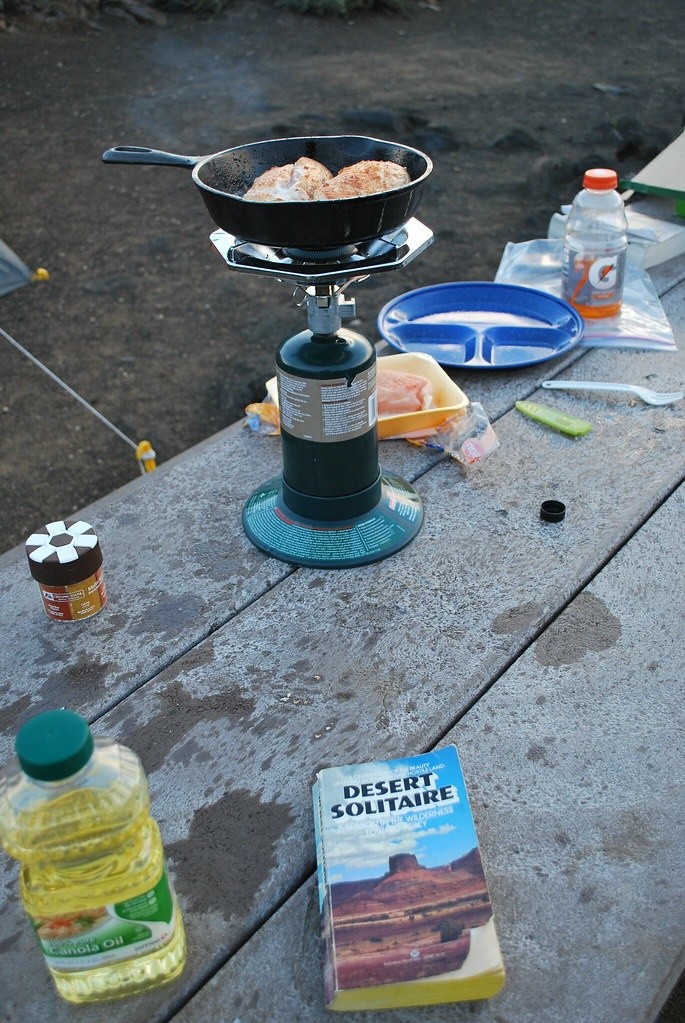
[[25, 520, 107, 622], [1, 709, 190, 1005], [560, 168, 629, 318]]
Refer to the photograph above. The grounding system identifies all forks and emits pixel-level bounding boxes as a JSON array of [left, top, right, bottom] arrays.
[[542, 378, 684, 405]]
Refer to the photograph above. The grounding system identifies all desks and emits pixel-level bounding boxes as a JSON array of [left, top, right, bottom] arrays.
[[1, 215, 685, 1021]]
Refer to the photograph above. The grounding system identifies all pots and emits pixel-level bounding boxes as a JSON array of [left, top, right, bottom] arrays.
[[101, 131, 434, 247]]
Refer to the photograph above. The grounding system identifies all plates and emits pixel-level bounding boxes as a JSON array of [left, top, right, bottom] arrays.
[[264, 352, 469, 443], [377, 279, 584, 370]]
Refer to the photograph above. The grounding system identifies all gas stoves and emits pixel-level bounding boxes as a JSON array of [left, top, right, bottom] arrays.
[[206, 214, 435, 566]]
